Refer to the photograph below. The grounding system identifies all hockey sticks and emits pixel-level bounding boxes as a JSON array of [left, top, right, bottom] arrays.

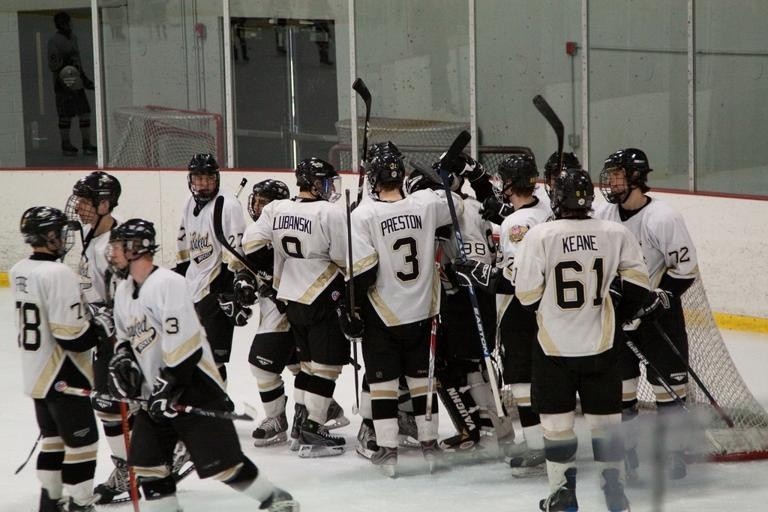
[[66, 220, 89, 279], [64, 387, 256, 422], [214, 195, 359, 373], [532, 93, 564, 177], [345, 189, 360, 415], [105, 263, 140, 512], [352, 77, 371, 206], [410, 155, 445, 423], [441, 130, 507, 420]]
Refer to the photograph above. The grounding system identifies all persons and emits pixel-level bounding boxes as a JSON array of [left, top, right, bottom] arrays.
[[105, 0, 127, 39], [110, 218, 303, 511], [169, 153, 259, 386], [308, 20, 335, 65], [240, 159, 350, 458], [443, 148, 696, 511], [63, 171, 122, 314], [271, 18, 294, 57], [9, 207, 115, 512], [341, 140, 464, 477], [48, 12, 98, 156], [229, 17, 250, 64], [151, 0, 169, 39]]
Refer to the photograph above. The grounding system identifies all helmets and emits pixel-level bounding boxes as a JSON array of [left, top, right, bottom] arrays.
[[20, 206, 75, 257], [62, 172, 121, 227], [106, 219, 156, 279]]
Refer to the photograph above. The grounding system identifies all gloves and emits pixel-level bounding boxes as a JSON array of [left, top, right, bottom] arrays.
[[332, 295, 366, 342], [620, 287, 672, 331], [83, 300, 190, 423], [215, 288, 252, 327], [233, 269, 256, 306], [449, 257, 503, 294]]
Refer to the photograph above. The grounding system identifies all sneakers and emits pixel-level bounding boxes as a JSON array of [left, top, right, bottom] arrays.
[[93, 455, 133, 503]]
